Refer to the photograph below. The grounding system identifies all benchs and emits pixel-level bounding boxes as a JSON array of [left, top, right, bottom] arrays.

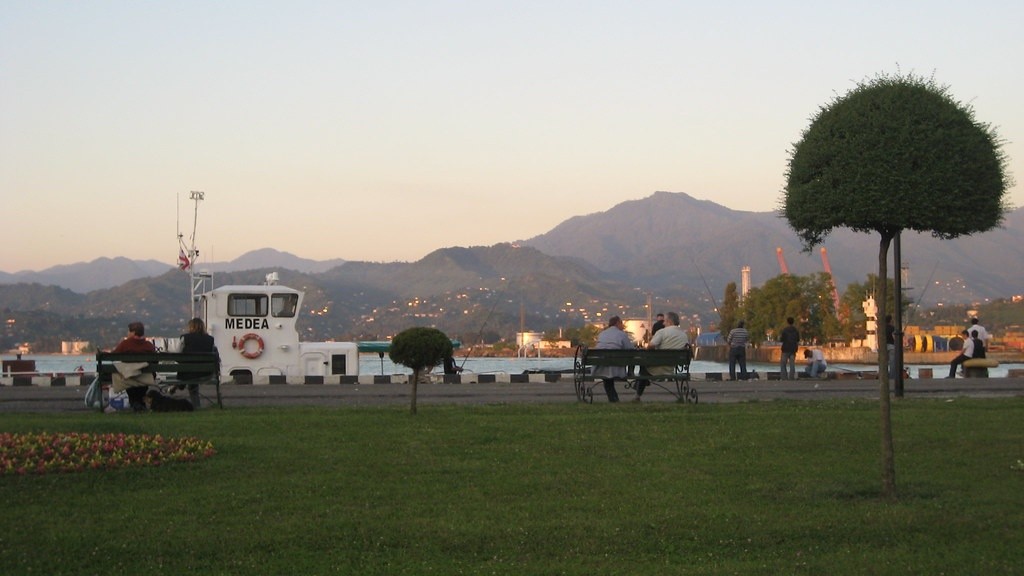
[[96, 345, 225, 411], [2, 371, 97, 387], [575, 342, 698, 405]]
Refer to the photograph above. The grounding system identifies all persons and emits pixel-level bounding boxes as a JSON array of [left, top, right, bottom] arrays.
[[885, 314, 894, 376], [804, 350, 827, 379], [168, 318, 218, 407], [591, 316, 634, 402], [107, 320, 158, 407], [778, 317, 800, 380], [944, 318, 988, 378], [632, 312, 689, 401], [726, 321, 749, 381]]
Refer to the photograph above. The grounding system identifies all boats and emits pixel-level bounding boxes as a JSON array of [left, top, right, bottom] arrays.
[[118, 190, 474, 387]]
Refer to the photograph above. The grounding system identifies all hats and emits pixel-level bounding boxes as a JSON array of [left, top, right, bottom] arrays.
[[804, 350, 809, 358]]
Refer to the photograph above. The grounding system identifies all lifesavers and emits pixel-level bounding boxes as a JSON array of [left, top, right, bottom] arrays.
[[239, 333, 265, 359]]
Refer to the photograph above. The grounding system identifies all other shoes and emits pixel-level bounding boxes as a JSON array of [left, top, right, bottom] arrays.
[[167, 387, 175, 394]]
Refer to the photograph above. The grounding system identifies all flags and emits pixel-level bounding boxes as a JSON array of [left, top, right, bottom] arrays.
[[176, 245, 190, 271]]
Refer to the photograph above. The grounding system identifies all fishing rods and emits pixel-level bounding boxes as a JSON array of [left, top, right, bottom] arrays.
[[690, 256, 730, 336], [901, 262, 940, 333], [456, 288, 505, 376]]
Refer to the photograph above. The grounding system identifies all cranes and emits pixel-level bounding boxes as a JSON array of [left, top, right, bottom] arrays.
[[820, 245, 849, 324], [775, 245, 811, 323]]
